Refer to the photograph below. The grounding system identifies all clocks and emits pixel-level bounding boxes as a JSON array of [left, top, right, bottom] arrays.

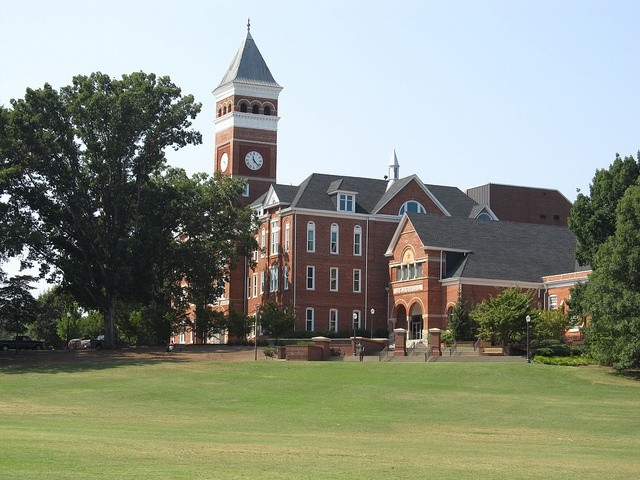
[[220, 152, 228, 172], [245, 150, 264, 171]]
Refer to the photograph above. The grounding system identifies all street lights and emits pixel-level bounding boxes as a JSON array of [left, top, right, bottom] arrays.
[[525, 314, 531, 362], [352, 313, 357, 356], [66, 311, 72, 346], [370, 308, 375, 338]]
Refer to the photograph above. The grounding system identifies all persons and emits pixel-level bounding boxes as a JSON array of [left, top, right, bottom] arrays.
[[170, 341, 174, 346]]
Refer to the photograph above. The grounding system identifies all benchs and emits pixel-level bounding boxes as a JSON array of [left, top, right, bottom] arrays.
[[481, 347, 504, 356]]
